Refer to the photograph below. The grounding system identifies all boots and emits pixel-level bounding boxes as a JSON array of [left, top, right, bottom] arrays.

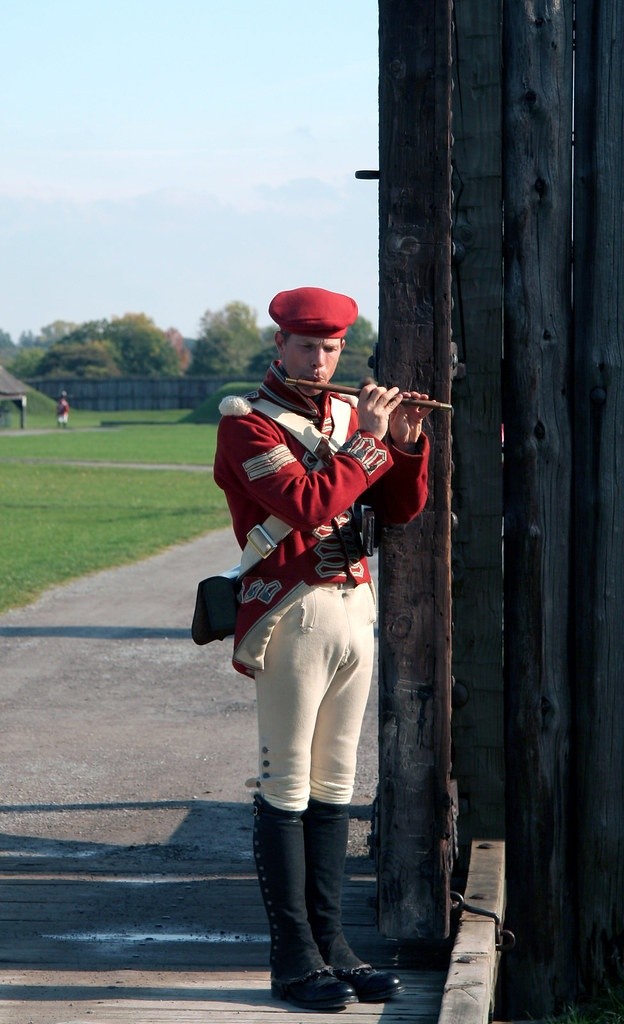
[[252, 793, 358, 1008], [302, 798, 406, 1000]]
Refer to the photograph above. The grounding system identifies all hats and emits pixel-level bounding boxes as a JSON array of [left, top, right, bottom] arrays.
[[269, 287, 359, 340]]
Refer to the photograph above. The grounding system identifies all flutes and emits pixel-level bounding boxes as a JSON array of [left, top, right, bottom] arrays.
[[283, 377, 451, 413]]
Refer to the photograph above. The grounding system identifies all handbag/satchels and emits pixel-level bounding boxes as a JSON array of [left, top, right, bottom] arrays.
[[192, 566, 240, 645]]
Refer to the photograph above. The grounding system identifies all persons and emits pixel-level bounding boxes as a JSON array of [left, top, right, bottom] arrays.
[[56, 391, 70, 429], [211, 287, 438, 1014]]
